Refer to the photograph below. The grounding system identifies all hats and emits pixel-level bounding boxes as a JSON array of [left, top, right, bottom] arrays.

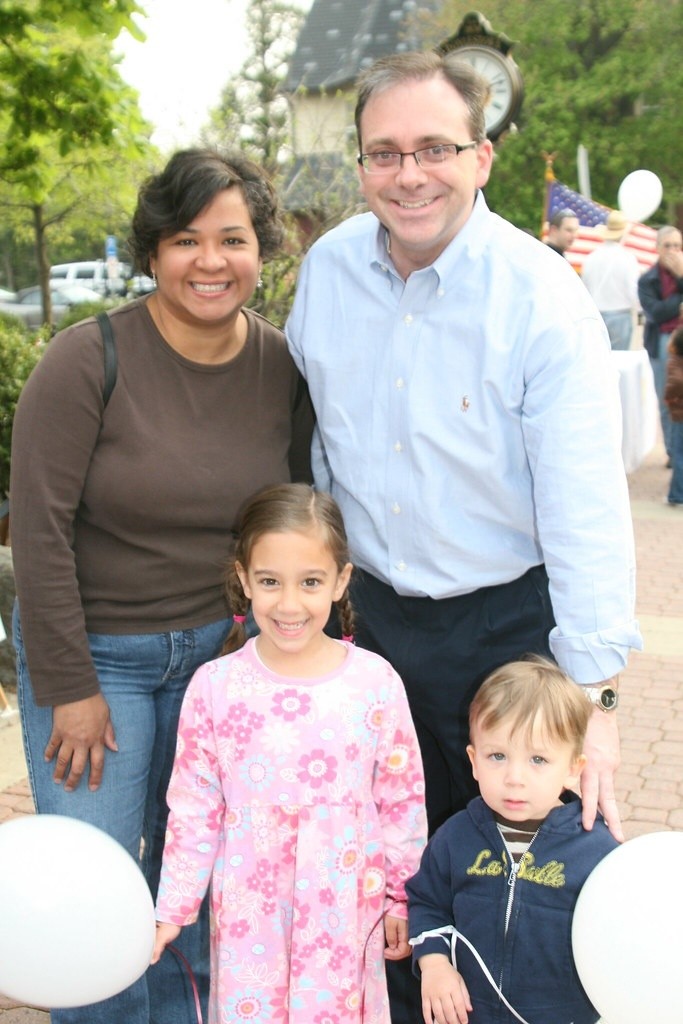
[[598, 210, 631, 240]]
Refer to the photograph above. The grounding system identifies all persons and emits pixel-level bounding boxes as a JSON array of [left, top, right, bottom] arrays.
[[281, 50, 641, 1024], [544, 208, 683, 509], [10, 140, 319, 1024], [407, 651, 623, 1024], [145, 482, 431, 1023]]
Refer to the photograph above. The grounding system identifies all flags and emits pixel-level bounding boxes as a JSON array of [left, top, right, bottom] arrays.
[[541, 172, 662, 311]]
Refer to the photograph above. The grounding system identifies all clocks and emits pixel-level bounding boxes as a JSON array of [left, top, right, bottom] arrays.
[[440, 45, 519, 136]]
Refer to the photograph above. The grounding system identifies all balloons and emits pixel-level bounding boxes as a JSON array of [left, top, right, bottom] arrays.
[[569, 831, 681, 1023], [1, 813, 159, 1011], [617, 169, 663, 226]]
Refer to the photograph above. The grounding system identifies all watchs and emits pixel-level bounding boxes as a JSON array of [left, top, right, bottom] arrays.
[[580, 682, 618, 712]]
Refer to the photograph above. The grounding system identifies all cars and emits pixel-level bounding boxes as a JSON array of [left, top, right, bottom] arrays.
[[0, 258, 157, 333]]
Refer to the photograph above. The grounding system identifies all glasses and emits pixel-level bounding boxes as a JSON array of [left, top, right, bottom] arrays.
[[356, 140, 478, 175]]
